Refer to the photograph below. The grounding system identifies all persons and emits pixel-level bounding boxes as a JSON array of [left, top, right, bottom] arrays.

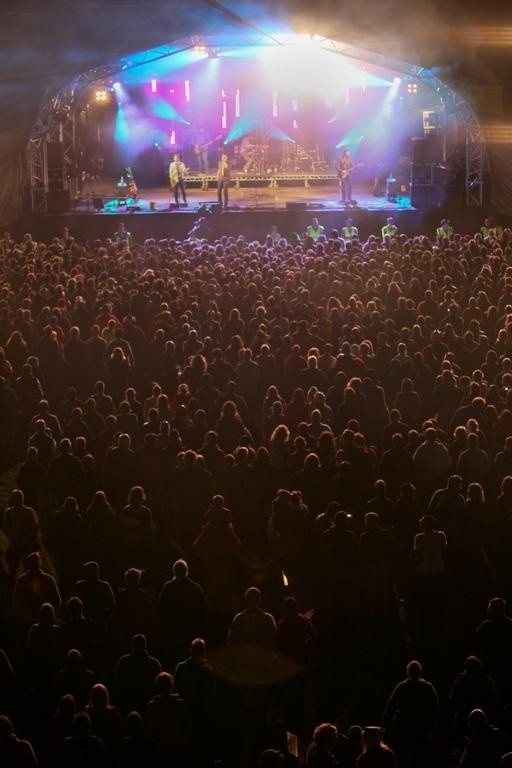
[[239, 132, 258, 173], [168, 152, 190, 205], [1, 214, 510, 767], [335, 147, 357, 201], [216, 153, 231, 208], [192, 126, 212, 173]]
